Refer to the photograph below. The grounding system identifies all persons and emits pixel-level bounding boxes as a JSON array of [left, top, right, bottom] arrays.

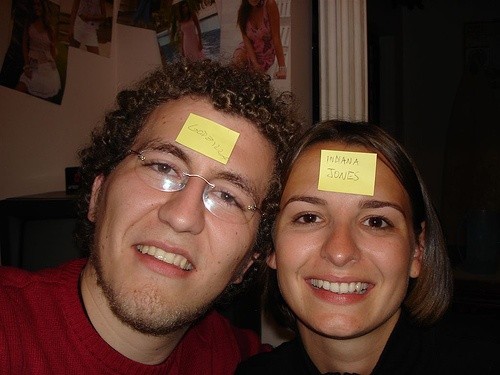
[[14, 0, 62, 99], [168, 1, 206, 66], [68, 0, 107, 55], [232, 119, 500, 375], [231, 0, 288, 80], [0, 57, 302, 375]]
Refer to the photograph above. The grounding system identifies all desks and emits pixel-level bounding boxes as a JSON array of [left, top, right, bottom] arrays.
[[0, 191, 79, 267]]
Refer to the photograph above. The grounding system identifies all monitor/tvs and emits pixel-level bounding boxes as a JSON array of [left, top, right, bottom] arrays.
[[4, 191, 85, 273]]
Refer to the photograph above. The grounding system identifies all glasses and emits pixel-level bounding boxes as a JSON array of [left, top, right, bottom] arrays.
[[129, 148, 265, 225]]
